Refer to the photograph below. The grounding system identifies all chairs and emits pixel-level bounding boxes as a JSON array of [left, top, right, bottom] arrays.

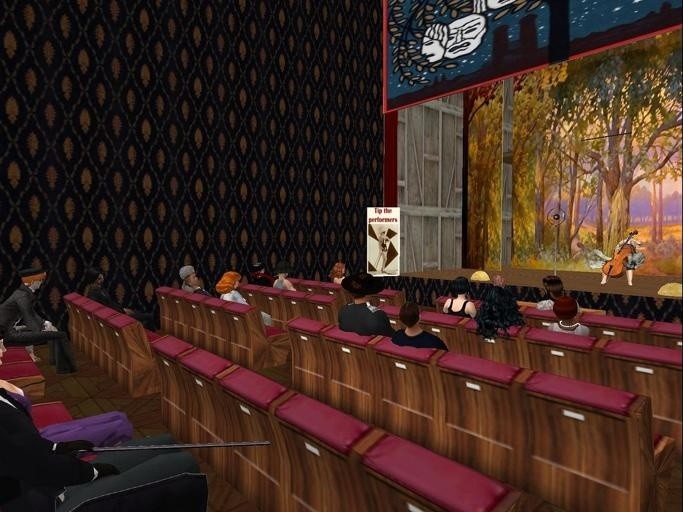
[[357, 430, 537, 512], [519, 368, 676, 511], [2, 267, 682, 461], [218, 365, 285, 510], [178, 346, 238, 479], [271, 391, 386, 510]]
